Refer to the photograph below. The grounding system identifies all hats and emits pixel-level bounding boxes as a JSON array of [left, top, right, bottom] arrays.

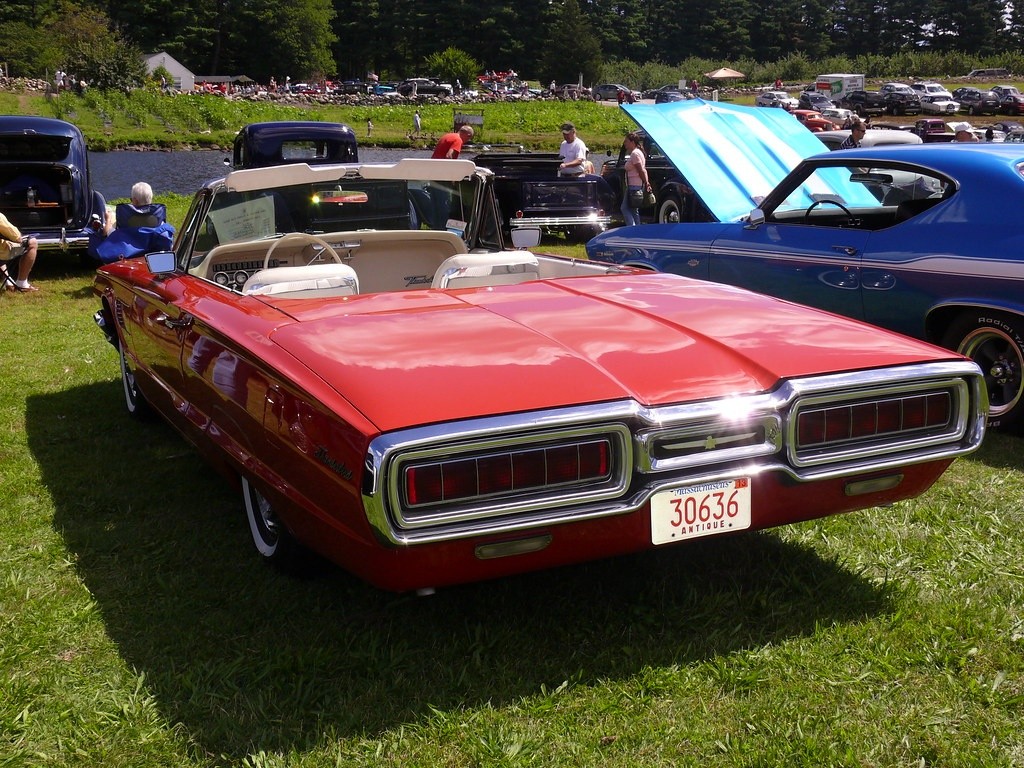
[[561, 123, 574, 134], [954, 122, 977, 133], [585, 148, 589, 152]]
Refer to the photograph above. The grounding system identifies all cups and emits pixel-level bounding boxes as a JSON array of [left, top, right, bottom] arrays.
[[60, 184, 71, 201]]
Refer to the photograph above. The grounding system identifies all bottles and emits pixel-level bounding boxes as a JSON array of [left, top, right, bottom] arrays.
[[648, 190, 656, 203], [27, 187, 36, 206]]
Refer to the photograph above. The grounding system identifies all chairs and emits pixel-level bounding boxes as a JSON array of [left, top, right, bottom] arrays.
[[431, 251, 541, 289], [242, 264, 359, 299], [894, 198, 944, 227]]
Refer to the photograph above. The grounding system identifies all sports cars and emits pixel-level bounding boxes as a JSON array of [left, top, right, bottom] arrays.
[[582, 96, 1024, 438], [91, 151, 992, 597]]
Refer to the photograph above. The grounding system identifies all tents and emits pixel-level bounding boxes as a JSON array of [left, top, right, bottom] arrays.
[[704, 67, 745, 88]]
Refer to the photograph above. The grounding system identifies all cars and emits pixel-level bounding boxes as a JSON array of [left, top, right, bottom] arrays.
[[89, 73, 1024, 248], [0, 113, 106, 268]]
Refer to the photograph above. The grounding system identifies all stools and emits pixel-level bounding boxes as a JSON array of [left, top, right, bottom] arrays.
[[0, 251, 27, 293]]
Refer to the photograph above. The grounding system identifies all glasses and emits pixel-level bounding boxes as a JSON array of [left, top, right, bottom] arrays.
[[857, 128, 865, 132], [130, 198, 136, 202]]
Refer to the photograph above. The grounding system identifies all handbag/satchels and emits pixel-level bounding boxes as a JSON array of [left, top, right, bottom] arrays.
[[627, 190, 644, 208]]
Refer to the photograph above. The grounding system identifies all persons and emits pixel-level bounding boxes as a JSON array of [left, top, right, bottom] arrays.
[[101, 182, 166, 241], [160, 75, 165, 89], [772, 98, 792, 111], [617, 89, 624, 104], [411, 110, 421, 137], [365, 118, 374, 138], [839, 122, 866, 176], [490, 77, 497, 94], [619, 132, 653, 226], [951, 122, 980, 142], [558, 123, 587, 178], [201, 76, 292, 94], [691, 80, 698, 93], [53, 68, 67, 94], [985, 126, 993, 142], [629, 90, 635, 104], [584, 160, 595, 175], [550, 81, 554, 94], [427, 125, 474, 231], [776, 78, 781, 86], [0, 211, 38, 292], [453, 78, 461, 93], [842, 110, 871, 128], [520, 80, 526, 96]]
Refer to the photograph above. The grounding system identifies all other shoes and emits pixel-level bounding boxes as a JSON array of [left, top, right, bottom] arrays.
[[15, 285, 38, 293], [6, 285, 16, 293]]
